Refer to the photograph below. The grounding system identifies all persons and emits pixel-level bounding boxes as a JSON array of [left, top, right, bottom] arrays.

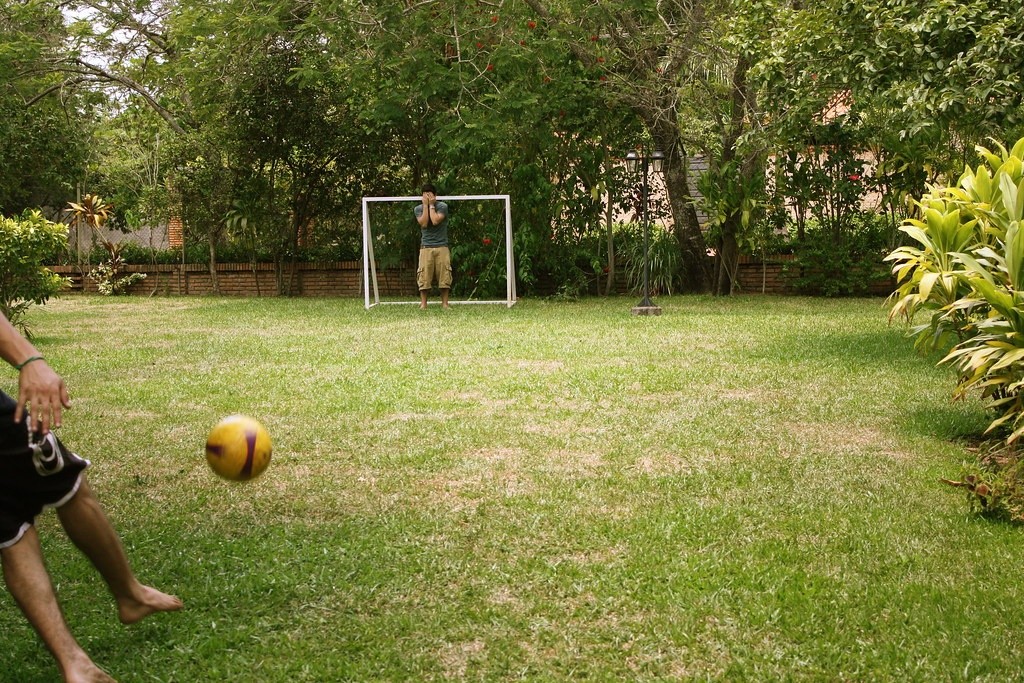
[[414, 184, 453, 311]]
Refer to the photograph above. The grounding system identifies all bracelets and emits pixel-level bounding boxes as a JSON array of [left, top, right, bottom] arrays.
[[0, 310, 183, 683], [17, 356, 46, 370], [429, 205, 436, 209]]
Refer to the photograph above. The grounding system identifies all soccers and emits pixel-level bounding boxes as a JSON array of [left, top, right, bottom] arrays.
[[205, 414, 274, 481]]
